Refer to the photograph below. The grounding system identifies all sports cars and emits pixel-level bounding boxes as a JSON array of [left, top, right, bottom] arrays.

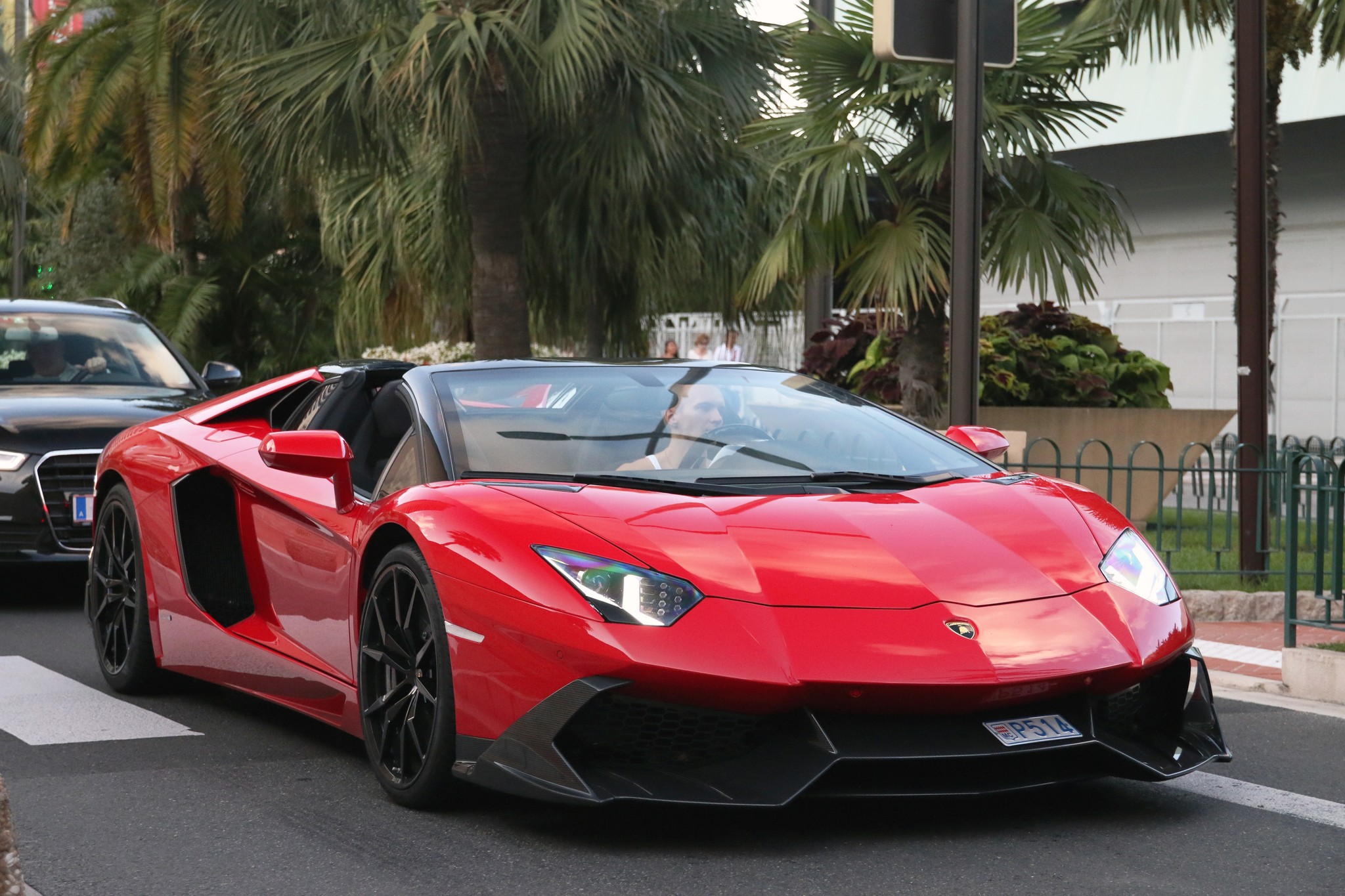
[[81, 356, 1237, 812]]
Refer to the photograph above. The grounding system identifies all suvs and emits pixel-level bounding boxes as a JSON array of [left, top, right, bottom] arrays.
[[0, 292, 247, 606]]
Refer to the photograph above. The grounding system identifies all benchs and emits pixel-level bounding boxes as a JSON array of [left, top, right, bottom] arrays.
[[372, 408, 666, 502]]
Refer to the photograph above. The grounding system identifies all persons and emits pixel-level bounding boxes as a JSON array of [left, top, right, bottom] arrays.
[[687, 333, 713, 360], [659, 340, 679, 359], [715, 329, 742, 362], [614, 385, 726, 471], [14, 337, 107, 383]]
